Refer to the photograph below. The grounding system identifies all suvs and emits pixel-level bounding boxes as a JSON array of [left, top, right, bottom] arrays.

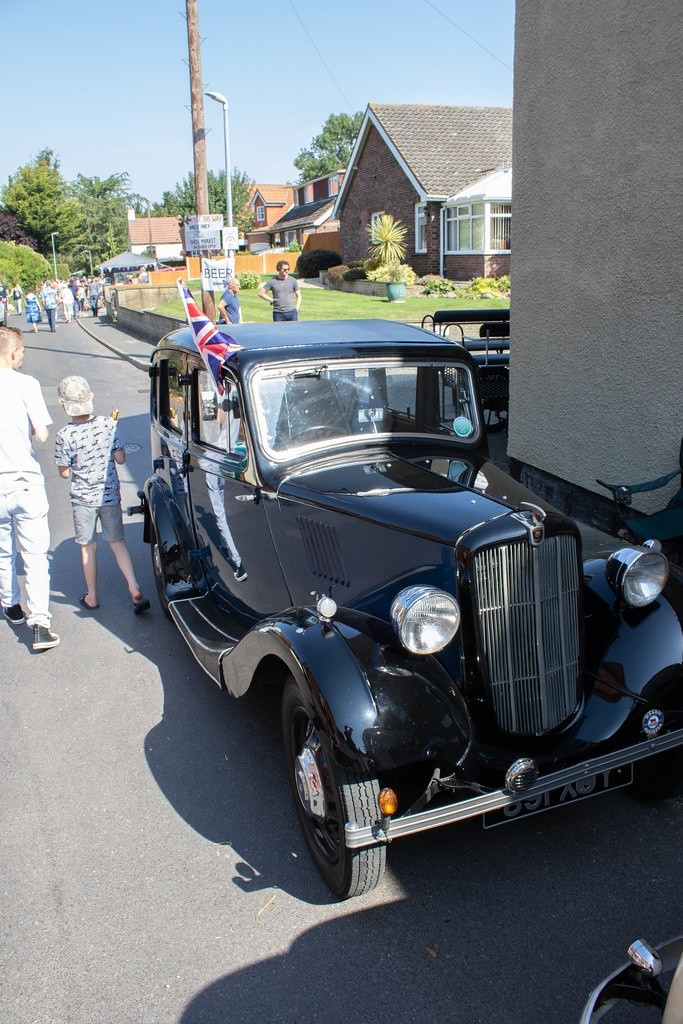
[[126, 319, 683, 893]]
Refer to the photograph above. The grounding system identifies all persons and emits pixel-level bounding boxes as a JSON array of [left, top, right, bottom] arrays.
[[54, 376, 151, 614], [0, 326, 60, 649], [258, 260, 301, 322], [217, 278, 242, 325], [0, 266, 149, 333], [168, 385, 248, 581]]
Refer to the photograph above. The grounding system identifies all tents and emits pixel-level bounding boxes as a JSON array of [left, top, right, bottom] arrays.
[[100, 251, 158, 278]]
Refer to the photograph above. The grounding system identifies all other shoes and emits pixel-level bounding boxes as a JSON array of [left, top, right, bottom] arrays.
[[3, 603, 25, 625], [32, 625, 61, 650]]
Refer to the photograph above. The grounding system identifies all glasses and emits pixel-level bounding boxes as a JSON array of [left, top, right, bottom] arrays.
[[281, 268, 289, 272]]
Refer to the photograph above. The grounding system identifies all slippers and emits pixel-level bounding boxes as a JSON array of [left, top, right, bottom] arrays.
[[79, 592, 100, 610], [133, 599, 150, 614]]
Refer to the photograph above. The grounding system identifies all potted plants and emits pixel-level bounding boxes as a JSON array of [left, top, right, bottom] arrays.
[[386, 270, 406, 303]]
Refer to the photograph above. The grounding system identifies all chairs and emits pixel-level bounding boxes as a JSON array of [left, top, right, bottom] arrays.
[[422, 308, 510, 425]]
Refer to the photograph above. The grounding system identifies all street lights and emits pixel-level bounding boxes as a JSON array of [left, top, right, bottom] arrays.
[[83, 251, 94, 276], [51, 232, 59, 280], [203, 91, 235, 283]]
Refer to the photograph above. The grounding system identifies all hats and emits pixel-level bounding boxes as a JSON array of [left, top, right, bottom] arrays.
[[57, 375, 95, 417]]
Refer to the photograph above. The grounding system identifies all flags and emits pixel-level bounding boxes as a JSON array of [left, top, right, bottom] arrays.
[[178, 279, 244, 396]]
